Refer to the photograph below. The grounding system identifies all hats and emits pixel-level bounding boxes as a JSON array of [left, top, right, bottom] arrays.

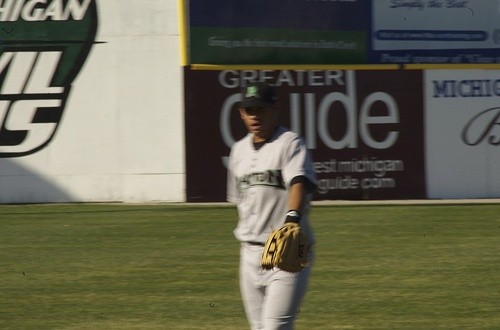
[[239, 83, 279, 108]]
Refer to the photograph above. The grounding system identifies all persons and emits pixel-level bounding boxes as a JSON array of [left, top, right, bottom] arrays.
[[226, 81, 318, 330]]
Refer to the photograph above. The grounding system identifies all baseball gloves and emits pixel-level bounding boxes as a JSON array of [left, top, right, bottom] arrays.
[[260, 222, 311, 273]]
